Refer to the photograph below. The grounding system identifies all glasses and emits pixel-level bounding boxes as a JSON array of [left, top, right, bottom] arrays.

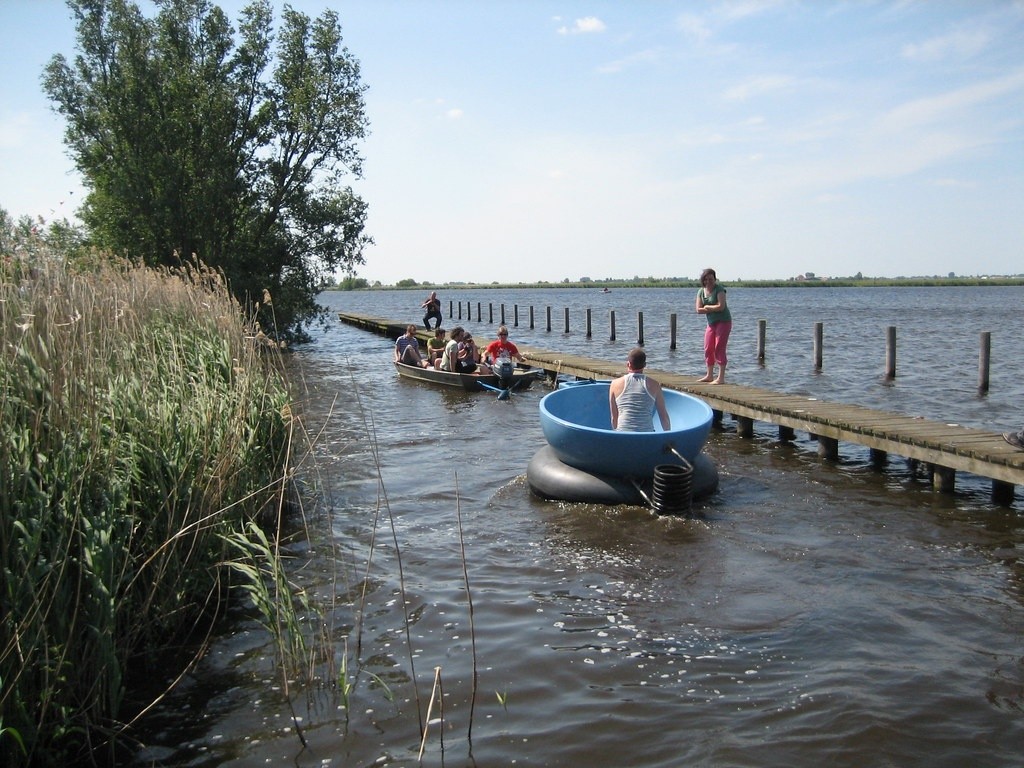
[[499, 333, 506, 336], [410, 331, 416, 335]]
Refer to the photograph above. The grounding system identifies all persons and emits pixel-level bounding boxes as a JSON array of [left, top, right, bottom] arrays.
[[395, 323, 430, 369], [609, 350, 671, 432], [696, 268, 732, 385], [481, 326, 526, 374], [421, 291, 443, 332], [427, 326, 479, 374]]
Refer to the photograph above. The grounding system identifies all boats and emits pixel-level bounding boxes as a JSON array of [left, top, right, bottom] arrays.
[[526, 378, 720, 515], [393, 357, 539, 391]]
